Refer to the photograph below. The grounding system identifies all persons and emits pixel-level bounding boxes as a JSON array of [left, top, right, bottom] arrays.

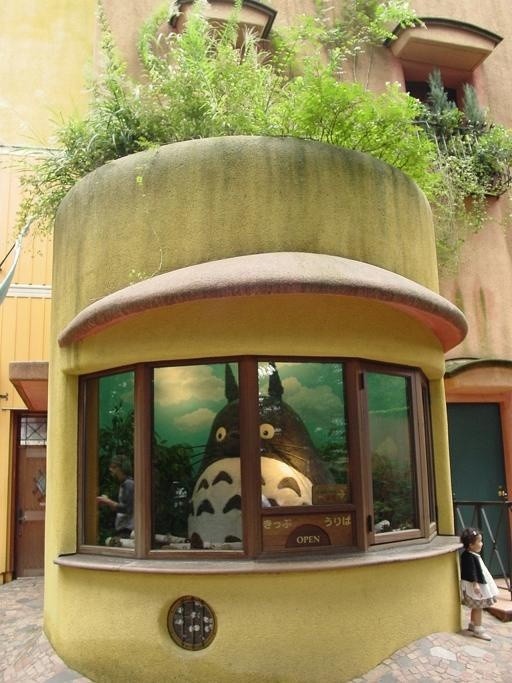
[[458, 526, 498, 642], [98, 454, 135, 539]]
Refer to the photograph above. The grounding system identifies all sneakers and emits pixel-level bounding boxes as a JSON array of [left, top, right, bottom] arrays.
[[468, 624, 492, 640]]
